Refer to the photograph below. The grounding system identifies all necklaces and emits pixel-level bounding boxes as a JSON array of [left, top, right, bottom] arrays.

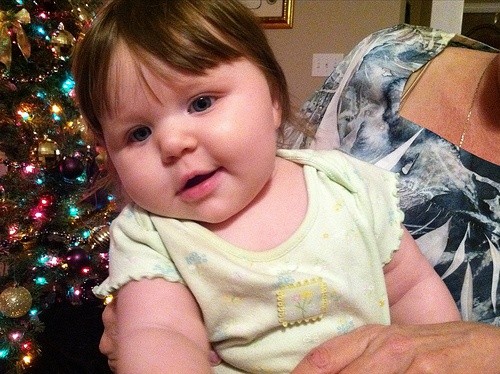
[[459, 54, 500, 147]]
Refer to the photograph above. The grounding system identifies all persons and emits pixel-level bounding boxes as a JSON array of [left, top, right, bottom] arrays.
[[73, 0, 500, 374]]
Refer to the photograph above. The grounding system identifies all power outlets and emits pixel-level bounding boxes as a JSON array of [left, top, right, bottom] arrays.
[[311, 53, 344, 77]]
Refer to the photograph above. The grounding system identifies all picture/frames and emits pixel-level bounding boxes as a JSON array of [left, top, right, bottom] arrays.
[[237, 0, 295, 30]]
